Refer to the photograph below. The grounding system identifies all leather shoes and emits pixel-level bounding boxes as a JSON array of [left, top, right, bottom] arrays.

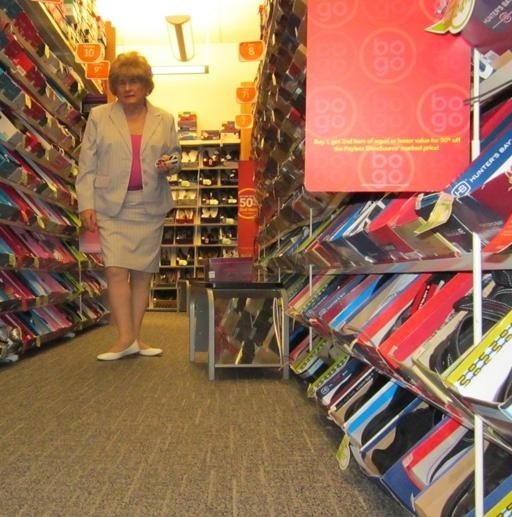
[[179, 149, 239, 168], [96, 338, 140, 361], [441, 441, 512, 517], [162, 169, 238, 265], [362, 384, 418, 446], [344, 369, 390, 422], [133, 347, 163, 356], [371, 405, 447, 475], [153, 267, 205, 288]]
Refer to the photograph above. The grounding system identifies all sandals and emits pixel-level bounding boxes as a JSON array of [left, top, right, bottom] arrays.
[[429, 270, 512, 375], [379, 271, 457, 348]]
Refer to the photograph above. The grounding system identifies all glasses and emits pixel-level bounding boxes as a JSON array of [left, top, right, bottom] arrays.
[[155, 155, 178, 168]]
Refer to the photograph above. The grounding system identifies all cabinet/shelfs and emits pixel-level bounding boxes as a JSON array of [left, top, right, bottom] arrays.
[[255, 2, 511, 516], [149, 138, 239, 310], [0, 0, 107, 364]]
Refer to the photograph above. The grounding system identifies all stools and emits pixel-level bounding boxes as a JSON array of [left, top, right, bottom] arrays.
[[183, 279, 291, 381]]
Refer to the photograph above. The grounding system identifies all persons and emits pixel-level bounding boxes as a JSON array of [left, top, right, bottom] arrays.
[[75, 50, 183, 362]]
